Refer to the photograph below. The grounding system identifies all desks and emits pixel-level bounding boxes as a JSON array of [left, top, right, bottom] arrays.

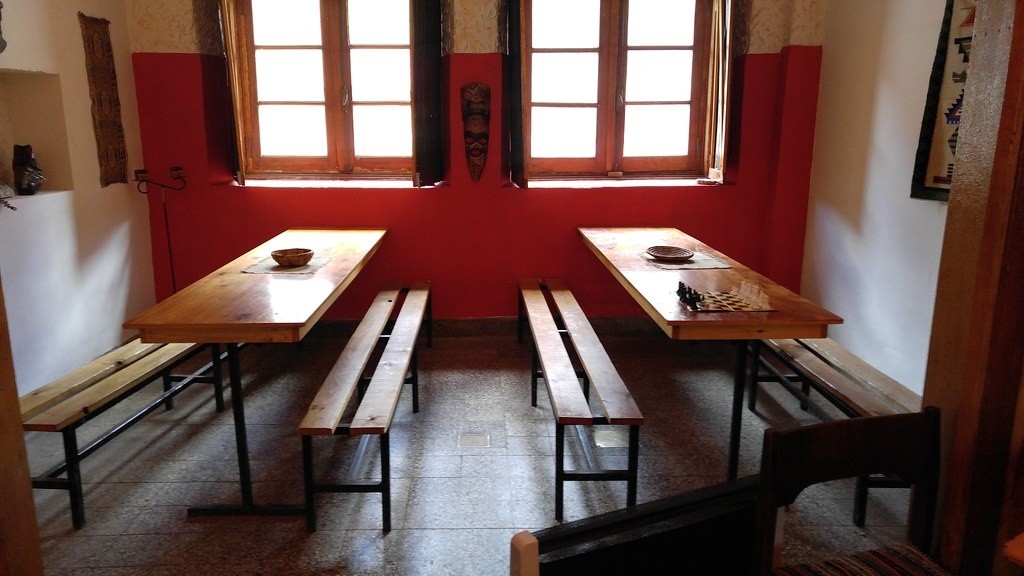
[[578, 227, 843, 483], [121, 226, 388, 518]]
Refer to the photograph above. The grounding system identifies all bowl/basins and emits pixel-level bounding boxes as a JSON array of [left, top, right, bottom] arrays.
[[271, 249, 314, 266]]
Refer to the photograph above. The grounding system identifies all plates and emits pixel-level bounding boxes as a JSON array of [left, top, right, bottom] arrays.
[[646, 245, 694, 261]]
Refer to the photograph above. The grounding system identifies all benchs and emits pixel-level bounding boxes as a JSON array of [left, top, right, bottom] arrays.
[[517, 276, 644, 521], [745, 339, 912, 527], [22, 338, 247, 530], [299, 278, 434, 532]]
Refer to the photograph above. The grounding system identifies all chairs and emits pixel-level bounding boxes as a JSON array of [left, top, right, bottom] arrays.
[[760, 405, 953, 576], [511, 470, 768, 576]]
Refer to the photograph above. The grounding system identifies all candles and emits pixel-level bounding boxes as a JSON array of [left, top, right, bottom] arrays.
[[169, 166, 183, 178], [134, 168, 149, 180]]
